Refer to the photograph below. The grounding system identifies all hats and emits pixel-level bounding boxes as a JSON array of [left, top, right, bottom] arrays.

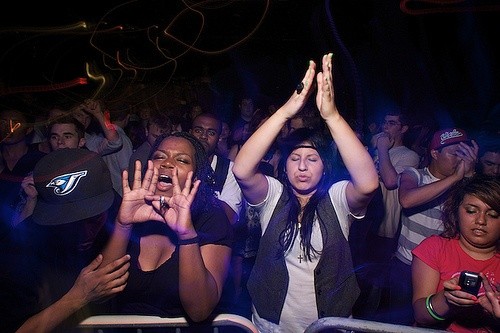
[[31, 148, 114, 226], [428, 129, 468, 150]]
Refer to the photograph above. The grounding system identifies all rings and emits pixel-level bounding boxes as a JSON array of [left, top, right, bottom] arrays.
[[297, 83, 304, 94]]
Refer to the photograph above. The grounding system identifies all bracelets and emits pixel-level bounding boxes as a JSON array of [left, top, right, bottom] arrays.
[[426, 294, 445, 321], [178, 235, 201, 244]]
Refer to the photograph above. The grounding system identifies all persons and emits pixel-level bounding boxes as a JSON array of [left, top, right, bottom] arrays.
[[411, 176, 500, 333], [380, 129, 500, 325], [96, 132, 232, 333], [233, 53, 379, 333], [192, 115, 242, 230], [0, 148, 131, 333], [371, 111, 421, 238], [0, 96, 325, 224]]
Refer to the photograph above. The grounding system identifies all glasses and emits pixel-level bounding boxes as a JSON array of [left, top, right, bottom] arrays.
[[382, 120, 400, 127]]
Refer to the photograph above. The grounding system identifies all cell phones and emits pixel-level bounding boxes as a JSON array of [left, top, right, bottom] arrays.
[[459, 271, 483, 296]]
